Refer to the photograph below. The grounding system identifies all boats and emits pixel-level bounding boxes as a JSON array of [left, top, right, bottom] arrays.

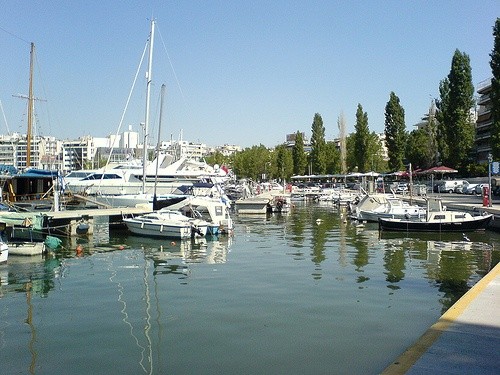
[[290, 188, 411, 218], [378, 211, 494, 232], [123, 210, 210, 239], [6, 241, 46, 256], [360, 204, 447, 222], [179, 201, 234, 234], [0, 236, 8, 262]]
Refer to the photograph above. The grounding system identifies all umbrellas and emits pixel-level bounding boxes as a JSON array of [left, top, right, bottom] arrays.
[[291, 165, 458, 187]]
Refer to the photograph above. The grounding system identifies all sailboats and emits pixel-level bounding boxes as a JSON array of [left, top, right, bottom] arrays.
[[0, 12, 291, 222]]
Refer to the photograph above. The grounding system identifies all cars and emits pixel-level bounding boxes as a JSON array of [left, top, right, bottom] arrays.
[[309, 182, 413, 193], [425, 180, 479, 194]]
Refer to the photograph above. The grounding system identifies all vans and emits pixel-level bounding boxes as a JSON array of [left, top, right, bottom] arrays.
[[466, 184, 491, 195]]
